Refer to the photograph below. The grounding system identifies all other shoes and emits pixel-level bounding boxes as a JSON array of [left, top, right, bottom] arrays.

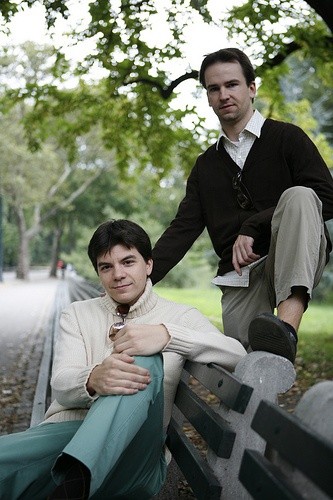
[[13, 467, 86, 500], [248, 311, 298, 365]]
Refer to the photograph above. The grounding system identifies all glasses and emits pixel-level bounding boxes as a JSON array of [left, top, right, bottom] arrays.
[[231, 169, 255, 209], [108, 303, 131, 341]]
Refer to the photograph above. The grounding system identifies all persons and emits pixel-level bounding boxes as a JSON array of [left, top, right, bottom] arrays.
[[0, 219, 248, 500], [55, 259, 73, 279], [151, 48, 333, 364]]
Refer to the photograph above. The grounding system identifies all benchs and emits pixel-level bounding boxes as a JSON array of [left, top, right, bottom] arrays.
[[30, 276, 254, 500], [238, 399, 333, 500]]
